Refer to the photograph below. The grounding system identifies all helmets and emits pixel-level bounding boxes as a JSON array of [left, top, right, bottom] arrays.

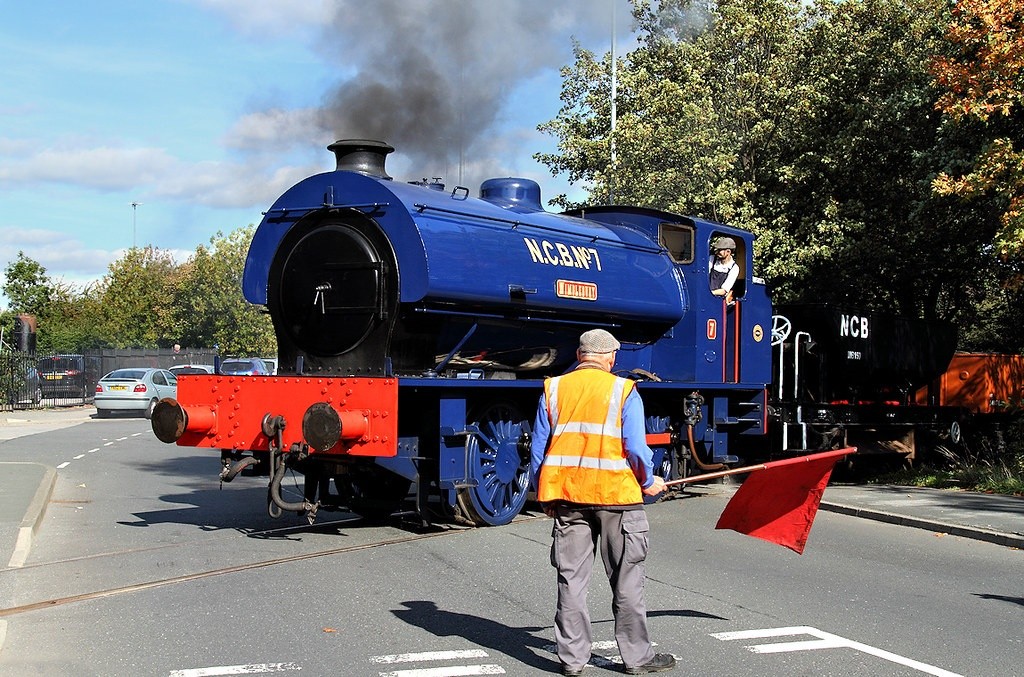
[[714, 238, 736, 249]]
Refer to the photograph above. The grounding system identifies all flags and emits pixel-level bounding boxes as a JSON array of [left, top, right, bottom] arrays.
[[714, 447, 858, 556]]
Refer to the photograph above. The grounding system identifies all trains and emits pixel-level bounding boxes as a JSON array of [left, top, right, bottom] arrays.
[[150, 139, 1024, 527]]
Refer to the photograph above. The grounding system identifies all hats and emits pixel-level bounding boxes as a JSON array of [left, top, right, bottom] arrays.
[[578, 329, 620, 353]]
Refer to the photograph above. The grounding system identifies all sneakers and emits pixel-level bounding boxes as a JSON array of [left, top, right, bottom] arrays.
[[562, 668, 583, 676], [625, 654, 676, 675]]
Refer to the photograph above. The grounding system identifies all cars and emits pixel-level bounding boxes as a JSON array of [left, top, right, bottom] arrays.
[[14, 355, 99, 403], [94, 356, 277, 418]]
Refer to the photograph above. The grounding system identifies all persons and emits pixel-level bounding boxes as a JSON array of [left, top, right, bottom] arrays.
[[531, 329, 674, 677], [708, 238, 740, 302]]
